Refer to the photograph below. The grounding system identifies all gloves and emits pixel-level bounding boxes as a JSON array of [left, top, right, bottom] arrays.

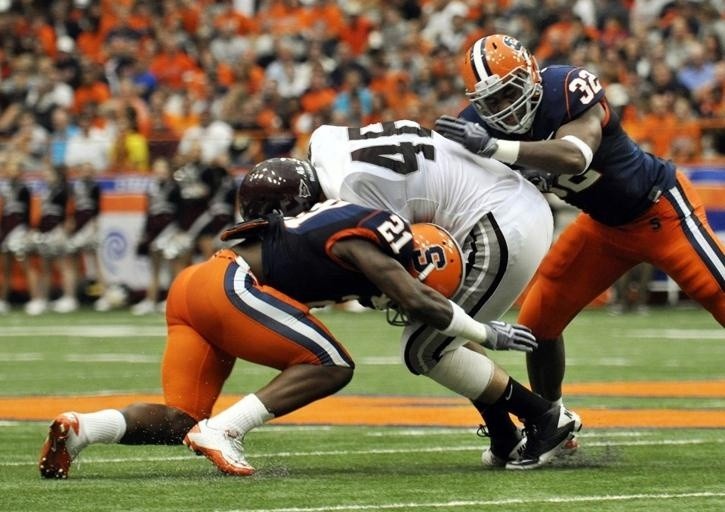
[[435, 114, 499, 157], [481, 320, 541, 354]]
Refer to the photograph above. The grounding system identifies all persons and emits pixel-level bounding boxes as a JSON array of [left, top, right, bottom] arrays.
[[42, 200, 540, 477], [1, 1, 725, 318], [433, 33, 725, 457]]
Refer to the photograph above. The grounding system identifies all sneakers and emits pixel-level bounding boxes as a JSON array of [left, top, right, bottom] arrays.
[[40, 411, 84, 480], [182, 418, 257, 476], [481, 402, 583, 472]]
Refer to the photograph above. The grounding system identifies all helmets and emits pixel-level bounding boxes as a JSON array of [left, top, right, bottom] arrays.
[[388, 223, 467, 326], [462, 34, 545, 135], [238, 157, 318, 223]]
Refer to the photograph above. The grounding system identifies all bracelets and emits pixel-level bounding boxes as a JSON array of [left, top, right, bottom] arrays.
[[240, 120, 581, 470], [488, 138, 519, 166], [468, 316, 489, 345]]
